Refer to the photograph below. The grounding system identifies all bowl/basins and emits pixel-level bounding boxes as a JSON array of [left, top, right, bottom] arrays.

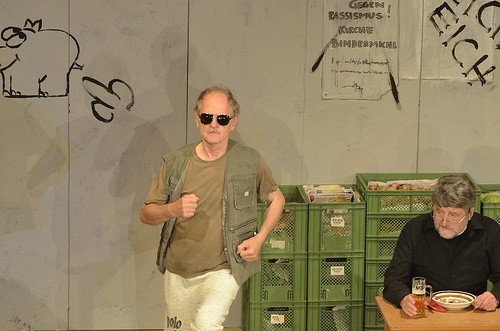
[[431, 290, 476, 311]]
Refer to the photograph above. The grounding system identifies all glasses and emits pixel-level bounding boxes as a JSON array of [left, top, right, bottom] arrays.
[[198, 112, 233, 126], [430, 210, 466, 225]]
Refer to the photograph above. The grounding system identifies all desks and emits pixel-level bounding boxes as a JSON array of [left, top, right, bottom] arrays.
[[375, 295, 500, 331]]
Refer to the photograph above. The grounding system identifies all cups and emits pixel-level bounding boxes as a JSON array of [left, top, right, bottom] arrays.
[[412, 277, 433, 318]]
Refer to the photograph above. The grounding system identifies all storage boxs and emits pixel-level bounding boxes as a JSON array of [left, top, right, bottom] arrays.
[[242, 173, 500, 331]]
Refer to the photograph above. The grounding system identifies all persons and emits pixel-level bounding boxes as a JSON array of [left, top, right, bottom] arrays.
[[382, 176, 500, 316], [138, 86, 286, 331]]
